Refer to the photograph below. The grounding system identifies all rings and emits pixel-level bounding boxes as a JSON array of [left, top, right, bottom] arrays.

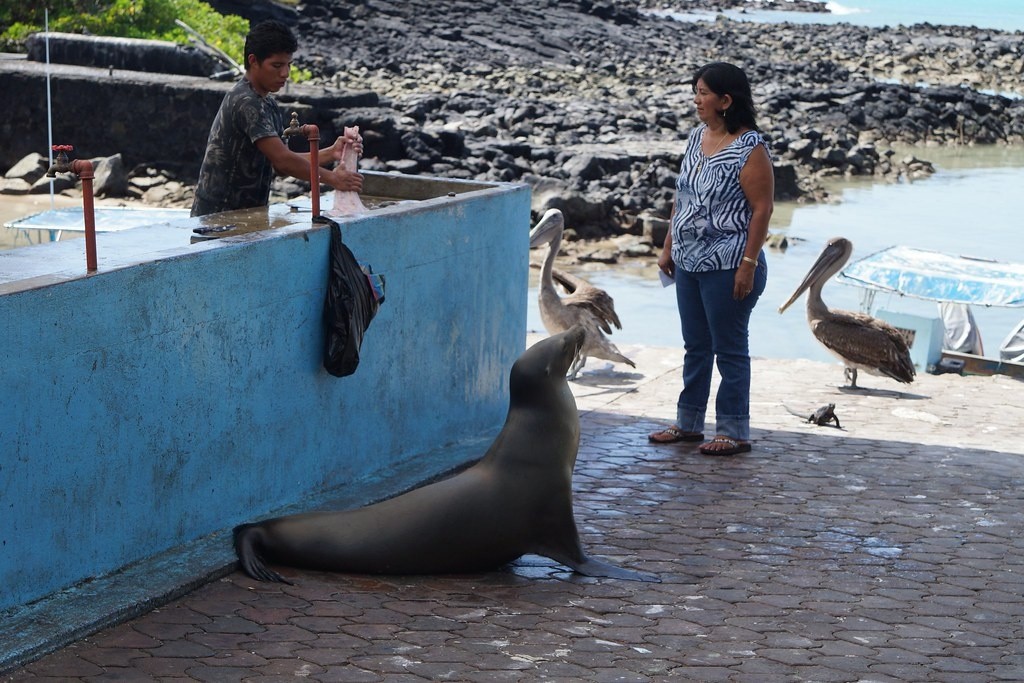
[[746, 289, 751, 293]]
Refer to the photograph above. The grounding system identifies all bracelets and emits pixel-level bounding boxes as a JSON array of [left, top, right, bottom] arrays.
[[742, 255, 759, 267]]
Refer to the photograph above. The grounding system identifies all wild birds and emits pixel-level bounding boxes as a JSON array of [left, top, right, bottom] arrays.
[[527, 208, 636, 382], [777, 236, 917, 391]]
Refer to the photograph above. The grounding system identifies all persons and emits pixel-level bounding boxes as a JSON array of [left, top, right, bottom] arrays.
[[188, 17, 366, 220], [646, 59, 776, 459]]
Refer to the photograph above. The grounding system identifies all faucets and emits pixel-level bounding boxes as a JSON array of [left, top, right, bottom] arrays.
[[44, 144, 73, 181], [280, 110, 305, 143]]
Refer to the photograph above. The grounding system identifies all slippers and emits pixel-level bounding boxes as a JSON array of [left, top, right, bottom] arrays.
[[648, 429, 704, 443], [700, 439, 751, 455]]
